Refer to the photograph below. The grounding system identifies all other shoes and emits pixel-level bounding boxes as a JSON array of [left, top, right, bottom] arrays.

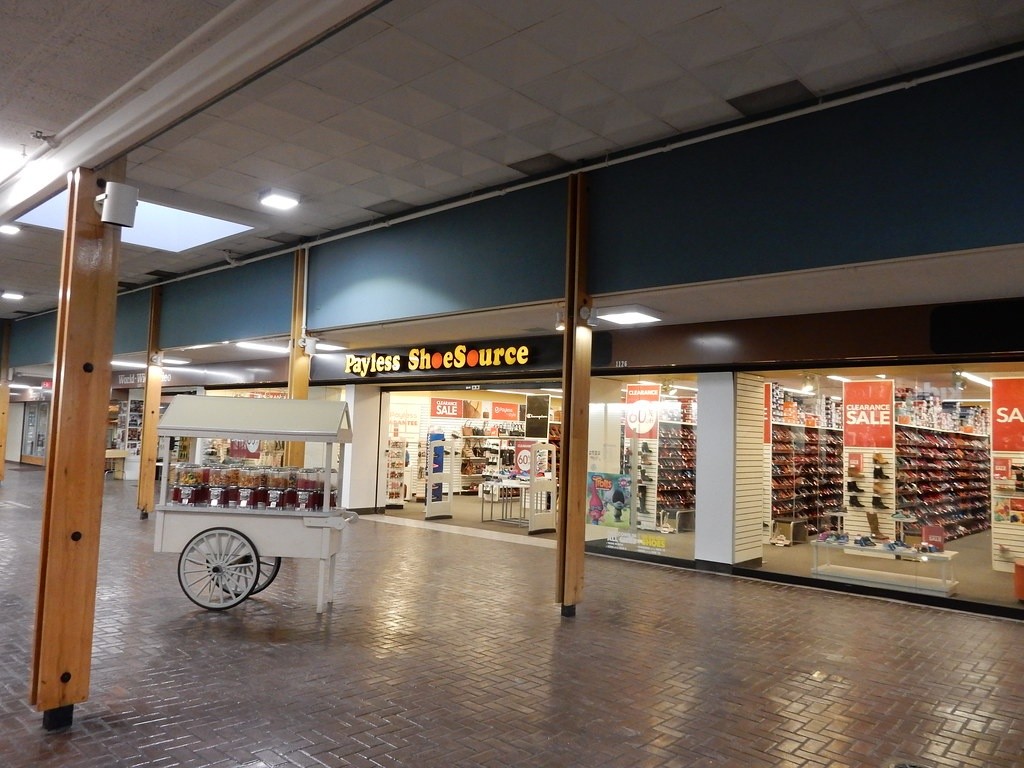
[[854, 536, 876, 547], [894, 540, 910, 548], [895, 427, 991, 543], [817, 530, 849, 544], [993, 465, 1024, 524], [772, 427, 843, 536], [657, 425, 697, 510], [888, 542, 897, 552]]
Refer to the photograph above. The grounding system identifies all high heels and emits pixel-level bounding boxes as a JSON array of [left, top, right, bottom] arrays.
[[848, 467, 864, 478], [872, 497, 889, 509], [849, 496, 865, 507], [848, 481, 864, 492], [872, 451, 888, 464], [873, 482, 888, 494], [874, 467, 889, 479], [641, 442, 652, 453]]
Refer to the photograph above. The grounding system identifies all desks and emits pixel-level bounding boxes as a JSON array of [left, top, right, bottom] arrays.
[[481, 479, 530, 528], [105, 449, 129, 481]]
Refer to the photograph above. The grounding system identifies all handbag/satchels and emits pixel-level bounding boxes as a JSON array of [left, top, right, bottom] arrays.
[[462, 418, 525, 476], [463, 399, 481, 418]]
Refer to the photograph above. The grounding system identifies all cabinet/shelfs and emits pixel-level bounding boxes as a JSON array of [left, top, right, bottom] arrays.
[[546, 421, 561, 513], [122, 389, 145, 480], [895, 422, 991, 542], [459, 435, 524, 495], [658, 420, 698, 519], [771, 421, 843, 527]]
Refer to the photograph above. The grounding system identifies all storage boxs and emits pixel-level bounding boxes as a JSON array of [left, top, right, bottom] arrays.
[[784, 402, 821, 426], [895, 392, 990, 434]]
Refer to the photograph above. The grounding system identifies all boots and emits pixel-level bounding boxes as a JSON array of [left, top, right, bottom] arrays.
[[641, 454, 652, 465], [866, 511, 889, 539], [641, 469, 653, 481], [637, 486, 649, 514], [999, 546, 1013, 560]]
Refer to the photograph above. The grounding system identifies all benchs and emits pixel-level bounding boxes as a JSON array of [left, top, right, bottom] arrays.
[[660, 508, 697, 534], [772, 517, 808, 547], [901, 524, 941, 562]]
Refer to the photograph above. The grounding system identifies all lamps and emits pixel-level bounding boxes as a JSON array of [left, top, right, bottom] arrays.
[[580, 307, 599, 326], [298, 336, 320, 355], [555, 311, 565, 331], [662, 379, 678, 396], [316, 339, 349, 351], [93, 182, 139, 228], [596, 304, 664, 325], [162, 356, 192, 364], [151, 350, 164, 367]]
